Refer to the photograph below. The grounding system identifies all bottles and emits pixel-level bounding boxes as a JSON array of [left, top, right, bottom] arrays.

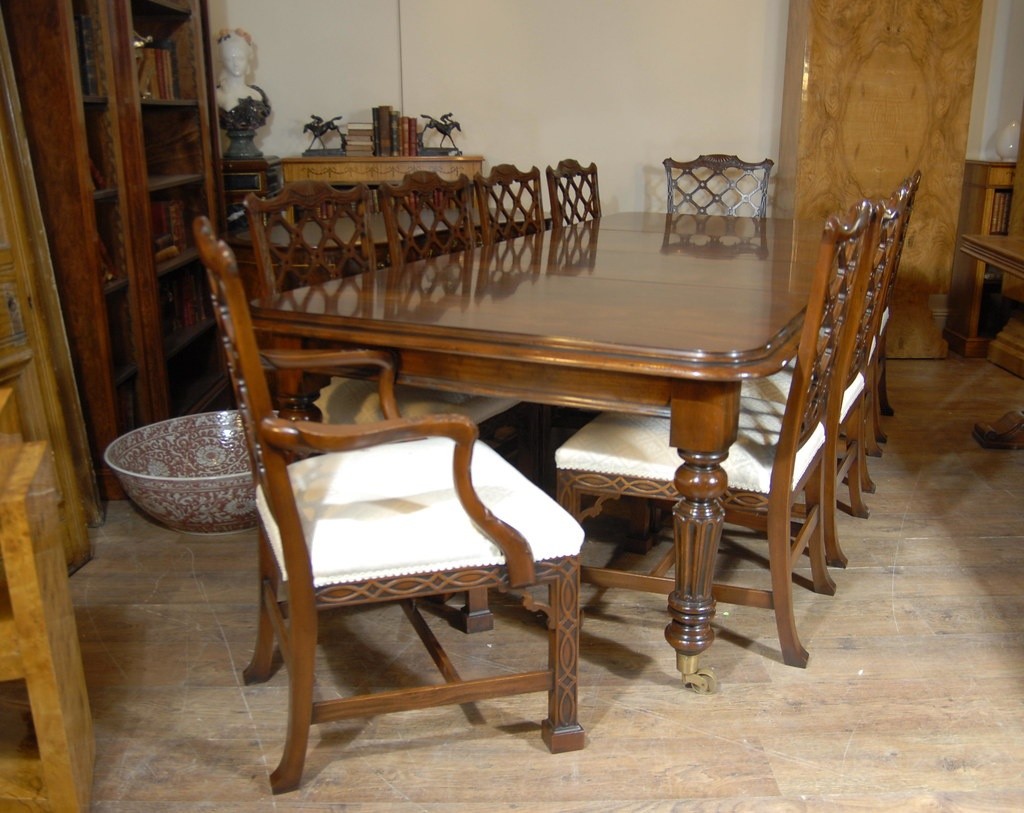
[[996, 119, 1021, 162]]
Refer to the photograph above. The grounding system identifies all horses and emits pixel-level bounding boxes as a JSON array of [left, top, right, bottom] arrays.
[[420, 114, 461, 148], [303, 116, 343, 152]]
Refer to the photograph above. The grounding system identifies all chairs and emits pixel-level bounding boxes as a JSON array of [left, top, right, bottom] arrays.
[[242, 154, 921, 671], [191, 216, 585, 794]]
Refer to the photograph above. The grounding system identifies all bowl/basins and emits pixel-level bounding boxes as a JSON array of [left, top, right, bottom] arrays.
[[104, 408, 279, 536]]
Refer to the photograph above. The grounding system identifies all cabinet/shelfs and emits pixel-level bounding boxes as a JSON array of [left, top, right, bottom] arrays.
[[2, 0, 231, 501], [943, 159, 1017, 359]]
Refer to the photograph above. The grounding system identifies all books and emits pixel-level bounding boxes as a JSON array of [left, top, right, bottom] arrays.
[[344, 105, 420, 158], [990, 191, 1009, 234], [131, 36, 198, 99], [73, 13, 135, 434], [151, 198, 187, 265], [171, 270, 207, 326]]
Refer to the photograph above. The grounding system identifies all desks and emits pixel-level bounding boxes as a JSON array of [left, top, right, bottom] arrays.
[[250, 212, 823, 695]]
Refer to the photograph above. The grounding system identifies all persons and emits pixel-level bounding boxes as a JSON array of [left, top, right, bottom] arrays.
[[215, 29, 264, 112]]
